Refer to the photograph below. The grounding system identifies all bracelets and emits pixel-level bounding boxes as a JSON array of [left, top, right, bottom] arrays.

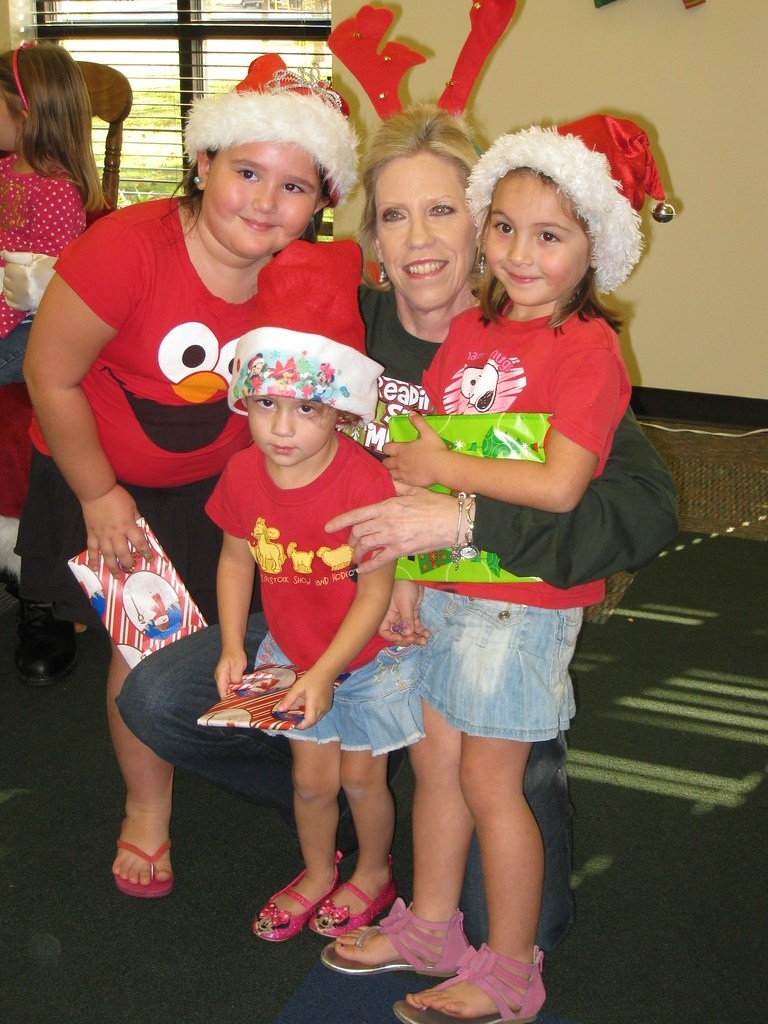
[[450, 487, 466, 574]]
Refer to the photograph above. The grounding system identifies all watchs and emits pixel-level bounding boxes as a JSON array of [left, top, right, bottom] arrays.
[[460, 494, 481, 564]]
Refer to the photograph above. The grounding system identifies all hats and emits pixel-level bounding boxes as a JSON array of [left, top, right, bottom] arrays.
[[182, 50, 362, 216], [225, 234, 385, 431], [464, 111, 675, 298]]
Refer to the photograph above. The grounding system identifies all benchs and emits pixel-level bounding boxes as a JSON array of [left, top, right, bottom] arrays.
[[550, 382, 768, 814]]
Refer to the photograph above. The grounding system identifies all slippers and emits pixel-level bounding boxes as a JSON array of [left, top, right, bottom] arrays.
[[114, 814, 175, 898]]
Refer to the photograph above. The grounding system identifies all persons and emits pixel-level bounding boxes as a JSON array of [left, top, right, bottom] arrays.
[[204, 232, 417, 949], [313, 109, 680, 1024], [118, 109, 678, 964], [0, 37, 110, 386], [13, 47, 370, 906]]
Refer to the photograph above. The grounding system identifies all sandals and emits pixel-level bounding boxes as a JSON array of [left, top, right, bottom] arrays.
[[391, 941, 546, 1024], [250, 845, 343, 941], [313, 893, 475, 977], [307, 856, 396, 940]]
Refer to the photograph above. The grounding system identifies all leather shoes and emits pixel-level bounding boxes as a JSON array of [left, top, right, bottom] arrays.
[[12, 596, 80, 686]]
